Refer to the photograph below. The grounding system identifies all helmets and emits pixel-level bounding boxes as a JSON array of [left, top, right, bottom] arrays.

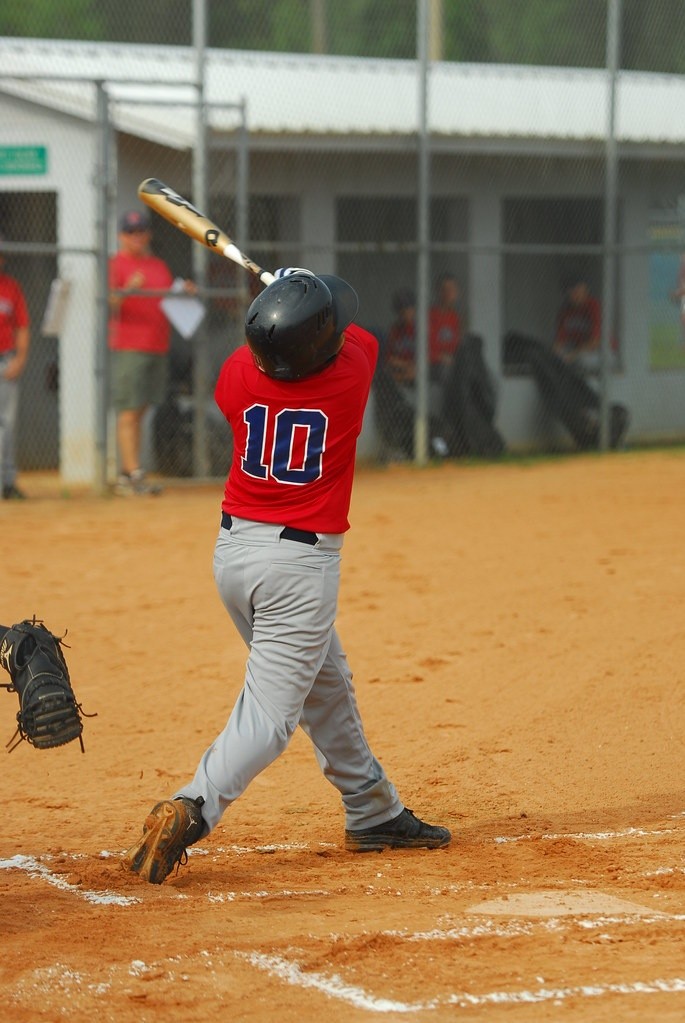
[[243, 268, 362, 386]]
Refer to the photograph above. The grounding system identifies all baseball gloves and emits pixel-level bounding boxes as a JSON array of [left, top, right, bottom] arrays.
[[0, 614, 96, 752]]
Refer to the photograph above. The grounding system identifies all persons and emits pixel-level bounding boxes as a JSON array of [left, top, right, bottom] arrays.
[[0, 234, 30, 503], [545, 273, 621, 371], [105, 207, 200, 498], [386, 290, 448, 461], [427, 271, 488, 457], [211, 229, 261, 322], [119, 265, 454, 880]]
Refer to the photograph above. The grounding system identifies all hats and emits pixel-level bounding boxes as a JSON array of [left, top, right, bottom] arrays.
[[116, 207, 153, 234]]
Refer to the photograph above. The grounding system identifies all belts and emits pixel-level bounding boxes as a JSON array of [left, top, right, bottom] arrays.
[[220, 511, 320, 547]]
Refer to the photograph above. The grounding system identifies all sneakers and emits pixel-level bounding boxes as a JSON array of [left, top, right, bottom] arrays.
[[118, 795, 207, 887], [342, 806, 452, 853]]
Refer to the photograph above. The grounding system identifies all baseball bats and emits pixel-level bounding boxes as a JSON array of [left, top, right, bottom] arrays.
[[137, 179, 274, 286]]
